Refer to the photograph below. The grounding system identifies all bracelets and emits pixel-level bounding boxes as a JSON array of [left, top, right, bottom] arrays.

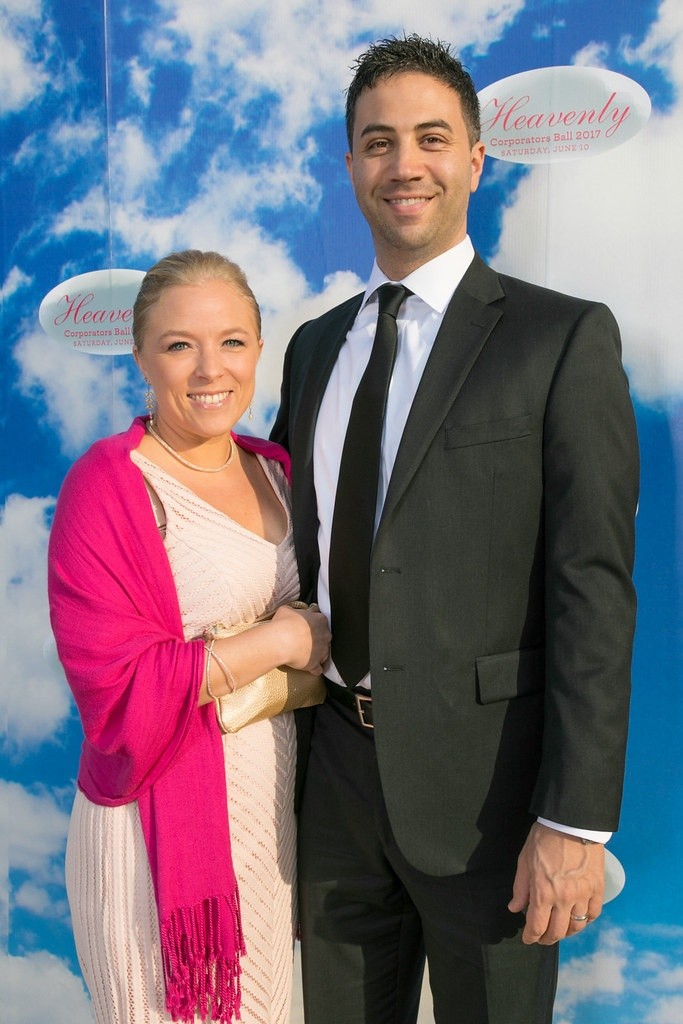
[[200, 641, 235, 699]]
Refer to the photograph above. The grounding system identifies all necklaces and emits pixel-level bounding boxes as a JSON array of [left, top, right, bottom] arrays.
[[144, 417, 238, 475]]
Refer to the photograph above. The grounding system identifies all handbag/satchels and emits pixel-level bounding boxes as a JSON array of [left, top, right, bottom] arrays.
[[202, 601, 326, 734]]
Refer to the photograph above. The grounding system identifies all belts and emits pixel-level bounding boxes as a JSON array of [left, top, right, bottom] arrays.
[[323, 675, 374, 729]]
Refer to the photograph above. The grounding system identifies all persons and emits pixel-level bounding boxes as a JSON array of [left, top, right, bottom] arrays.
[[47, 250, 333, 1024], [269, 34, 641, 1024]]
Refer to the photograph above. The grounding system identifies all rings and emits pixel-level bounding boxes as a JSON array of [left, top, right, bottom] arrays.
[[571, 914, 589, 921]]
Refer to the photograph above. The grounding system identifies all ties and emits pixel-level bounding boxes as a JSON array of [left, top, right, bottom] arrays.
[[329, 283, 414, 692]]
